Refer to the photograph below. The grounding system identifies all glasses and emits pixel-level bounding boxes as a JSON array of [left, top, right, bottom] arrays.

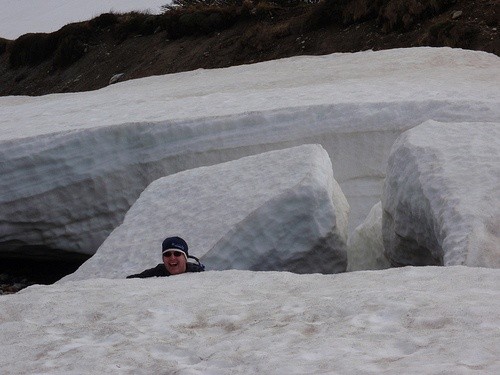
[[163, 251, 183, 257]]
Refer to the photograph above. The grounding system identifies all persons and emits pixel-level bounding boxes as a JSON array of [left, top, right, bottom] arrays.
[[126, 235, 206, 280]]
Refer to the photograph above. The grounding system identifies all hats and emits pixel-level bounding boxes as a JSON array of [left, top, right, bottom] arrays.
[[161, 236, 188, 260]]
